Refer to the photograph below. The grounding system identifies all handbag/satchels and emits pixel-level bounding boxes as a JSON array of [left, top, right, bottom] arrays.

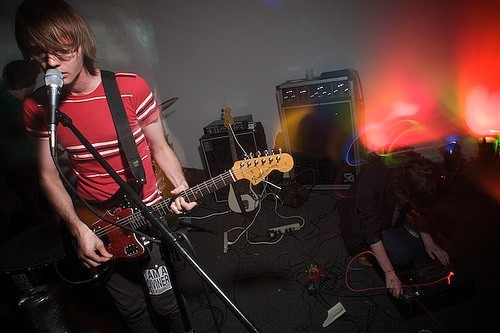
[[272, 169, 320, 208]]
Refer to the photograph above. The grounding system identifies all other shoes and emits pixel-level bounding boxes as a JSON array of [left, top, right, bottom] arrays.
[[356, 254, 377, 267]]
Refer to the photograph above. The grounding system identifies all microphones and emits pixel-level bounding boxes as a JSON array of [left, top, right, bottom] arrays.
[[45, 69, 64, 157]]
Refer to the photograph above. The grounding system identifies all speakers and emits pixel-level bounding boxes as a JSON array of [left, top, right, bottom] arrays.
[[283, 98, 366, 190], [200, 122, 273, 202]]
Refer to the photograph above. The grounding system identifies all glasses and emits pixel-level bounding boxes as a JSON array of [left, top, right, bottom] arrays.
[[30, 43, 80, 63]]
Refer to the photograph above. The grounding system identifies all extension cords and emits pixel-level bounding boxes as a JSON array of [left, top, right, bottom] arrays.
[[269, 223, 301, 234], [224, 232, 228, 253]]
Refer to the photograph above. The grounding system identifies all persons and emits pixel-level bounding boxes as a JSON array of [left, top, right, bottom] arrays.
[[14, 1, 199, 333], [0, 60, 69, 218]]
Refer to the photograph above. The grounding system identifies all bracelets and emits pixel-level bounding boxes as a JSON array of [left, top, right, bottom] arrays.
[[336, 144, 450, 299], [383, 269, 395, 273]]
